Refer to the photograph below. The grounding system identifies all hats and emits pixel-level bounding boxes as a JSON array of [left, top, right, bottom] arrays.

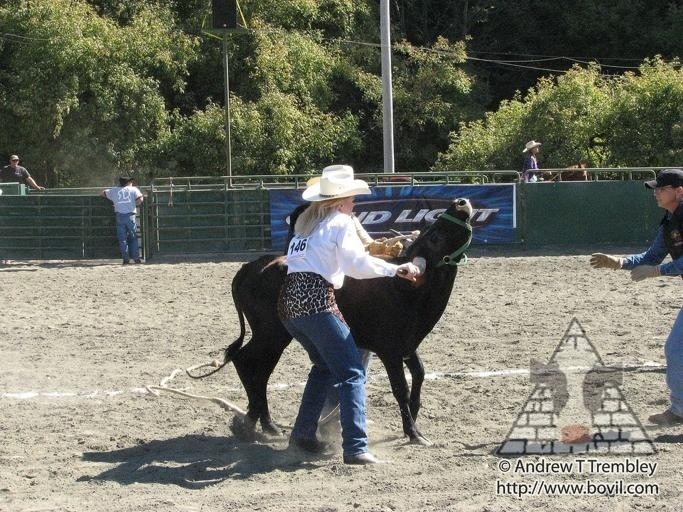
[[644, 169, 683, 189], [10, 154, 19, 160], [522, 141, 542, 153], [119, 177, 134, 187], [302, 164, 371, 202]]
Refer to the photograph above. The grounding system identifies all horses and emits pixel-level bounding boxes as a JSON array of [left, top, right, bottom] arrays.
[[544, 163, 589, 181]]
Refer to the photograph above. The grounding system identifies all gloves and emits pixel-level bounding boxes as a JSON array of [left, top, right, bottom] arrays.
[[589, 252, 624, 271], [368, 237, 387, 257], [630, 265, 661, 282], [386, 242, 403, 257]]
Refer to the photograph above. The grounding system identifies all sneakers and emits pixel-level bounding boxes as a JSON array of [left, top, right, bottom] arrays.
[[135, 259, 141, 263], [648, 411, 683, 424], [123, 260, 129, 263], [343, 452, 382, 464], [288, 439, 331, 452]]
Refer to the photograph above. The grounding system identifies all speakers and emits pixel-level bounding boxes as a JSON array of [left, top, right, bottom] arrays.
[[213, 2, 237, 30]]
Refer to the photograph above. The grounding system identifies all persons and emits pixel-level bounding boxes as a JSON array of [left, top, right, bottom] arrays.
[[277, 173, 420, 465], [590, 168, 683, 423], [101, 177, 144, 265], [523, 140, 551, 182], [0, 154, 46, 191], [286, 165, 401, 425]]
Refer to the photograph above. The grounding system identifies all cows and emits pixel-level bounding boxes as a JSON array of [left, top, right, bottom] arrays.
[[529, 348, 623, 443], [223, 197, 473, 448]]
